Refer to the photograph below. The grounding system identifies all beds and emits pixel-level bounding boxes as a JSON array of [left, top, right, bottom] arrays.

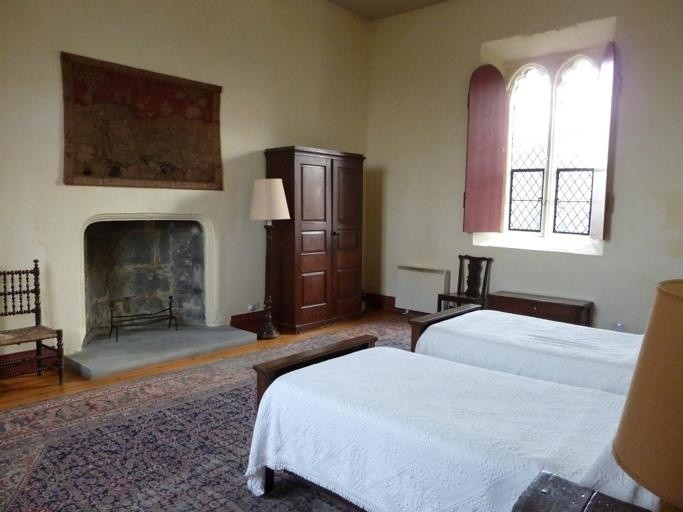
[[407, 303, 644, 396], [244, 335, 660, 512]]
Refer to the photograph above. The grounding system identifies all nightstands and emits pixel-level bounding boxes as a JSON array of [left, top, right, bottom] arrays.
[[508, 471, 645, 512]]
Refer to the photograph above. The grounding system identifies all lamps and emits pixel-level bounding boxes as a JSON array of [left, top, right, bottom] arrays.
[[611, 279, 683, 511], [247, 179, 291, 340]]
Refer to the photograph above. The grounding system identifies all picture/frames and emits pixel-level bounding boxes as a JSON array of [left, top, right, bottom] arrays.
[[61, 52, 223, 192]]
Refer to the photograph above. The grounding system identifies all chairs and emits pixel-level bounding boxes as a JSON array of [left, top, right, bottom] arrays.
[[0, 260, 63, 386], [437, 255, 495, 311]]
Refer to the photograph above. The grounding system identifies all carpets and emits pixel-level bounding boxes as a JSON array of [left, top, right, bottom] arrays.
[[0, 312, 414, 511]]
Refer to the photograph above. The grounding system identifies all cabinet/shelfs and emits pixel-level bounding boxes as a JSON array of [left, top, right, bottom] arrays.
[[488, 290, 594, 327], [265, 145, 367, 335]]
